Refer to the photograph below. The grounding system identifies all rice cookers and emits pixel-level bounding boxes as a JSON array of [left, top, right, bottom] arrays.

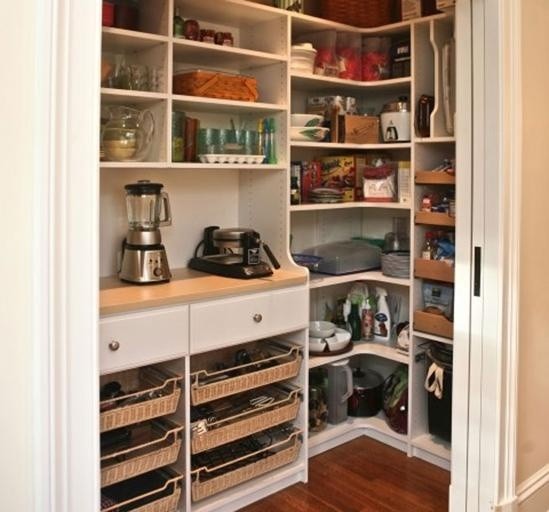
[[379, 95, 410, 143]]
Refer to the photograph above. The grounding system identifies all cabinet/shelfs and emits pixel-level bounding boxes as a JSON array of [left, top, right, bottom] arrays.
[[188, 329, 308, 512], [101, 3, 291, 166], [289, 12, 414, 457], [101, 353, 190, 511], [188, 282, 311, 353], [100, 303, 189, 375], [410, 11, 457, 475]]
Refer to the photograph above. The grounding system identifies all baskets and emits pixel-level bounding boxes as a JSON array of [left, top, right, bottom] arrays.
[[172, 68, 261, 103], [320, 0, 402, 29]]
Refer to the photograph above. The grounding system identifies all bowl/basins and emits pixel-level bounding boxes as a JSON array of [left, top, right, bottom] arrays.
[[308, 320, 336, 339], [290, 113, 325, 127], [290, 126, 332, 142]]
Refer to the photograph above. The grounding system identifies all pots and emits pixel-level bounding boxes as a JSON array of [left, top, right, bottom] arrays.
[[187, 225, 281, 281], [347, 365, 385, 417]]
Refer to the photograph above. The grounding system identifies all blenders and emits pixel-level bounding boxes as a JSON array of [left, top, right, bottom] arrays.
[[115, 180, 173, 286]]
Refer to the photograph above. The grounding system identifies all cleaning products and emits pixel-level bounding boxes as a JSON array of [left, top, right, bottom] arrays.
[[373, 287, 392, 340]]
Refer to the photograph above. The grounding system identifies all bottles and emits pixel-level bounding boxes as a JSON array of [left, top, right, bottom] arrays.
[[420, 231, 437, 260], [361, 297, 374, 341], [173, 6, 185, 39], [347, 299, 362, 341]]
